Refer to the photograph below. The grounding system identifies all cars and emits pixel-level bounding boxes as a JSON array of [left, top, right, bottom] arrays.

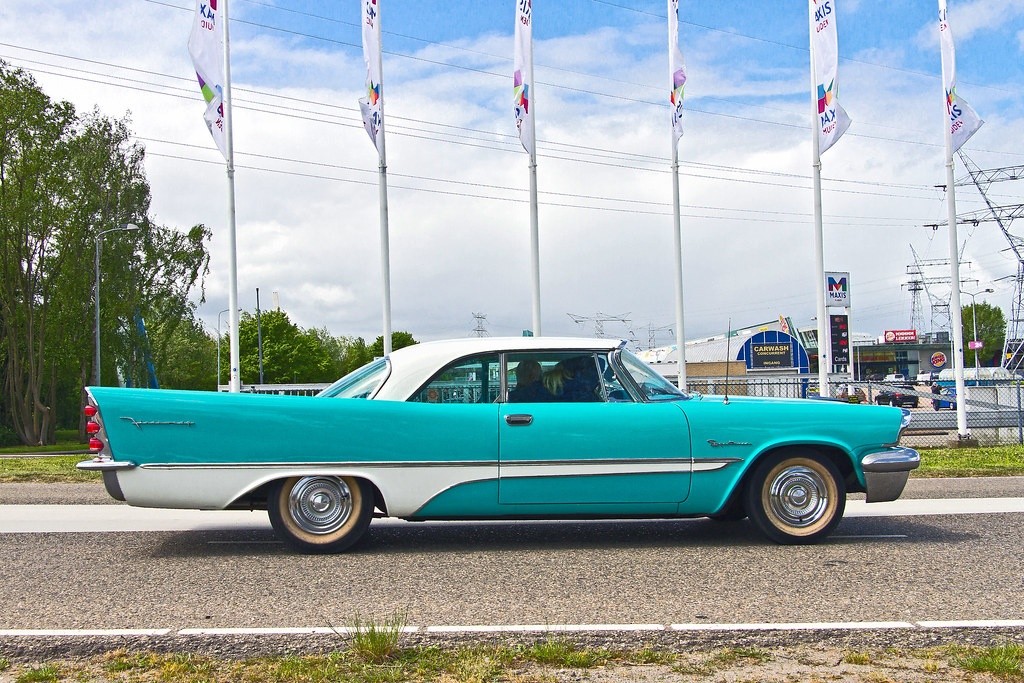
[[806, 377, 820, 399], [931, 388, 957, 411], [75, 335, 922, 553]]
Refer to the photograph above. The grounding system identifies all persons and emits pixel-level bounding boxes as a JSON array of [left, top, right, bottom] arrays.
[[508, 360, 576, 404], [930, 382, 943, 412]]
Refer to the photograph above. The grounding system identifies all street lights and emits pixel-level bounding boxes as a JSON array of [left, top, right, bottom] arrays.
[[95, 223, 140, 386], [959, 289, 995, 386], [216, 307, 242, 386], [255, 287, 265, 384]]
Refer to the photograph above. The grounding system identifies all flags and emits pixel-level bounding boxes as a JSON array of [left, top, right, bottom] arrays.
[[814, 0, 852, 155], [939, 1, 985, 155], [358, 0, 380, 160], [512, 1, 533, 156], [186, 0, 227, 161], [668, 0, 686, 153]]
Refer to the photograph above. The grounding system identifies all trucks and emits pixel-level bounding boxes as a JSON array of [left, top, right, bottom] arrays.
[[882, 374, 905, 383]]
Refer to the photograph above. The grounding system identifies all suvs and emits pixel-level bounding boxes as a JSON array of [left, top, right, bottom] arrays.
[[874, 384, 919, 408], [841, 387, 868, 403]]
